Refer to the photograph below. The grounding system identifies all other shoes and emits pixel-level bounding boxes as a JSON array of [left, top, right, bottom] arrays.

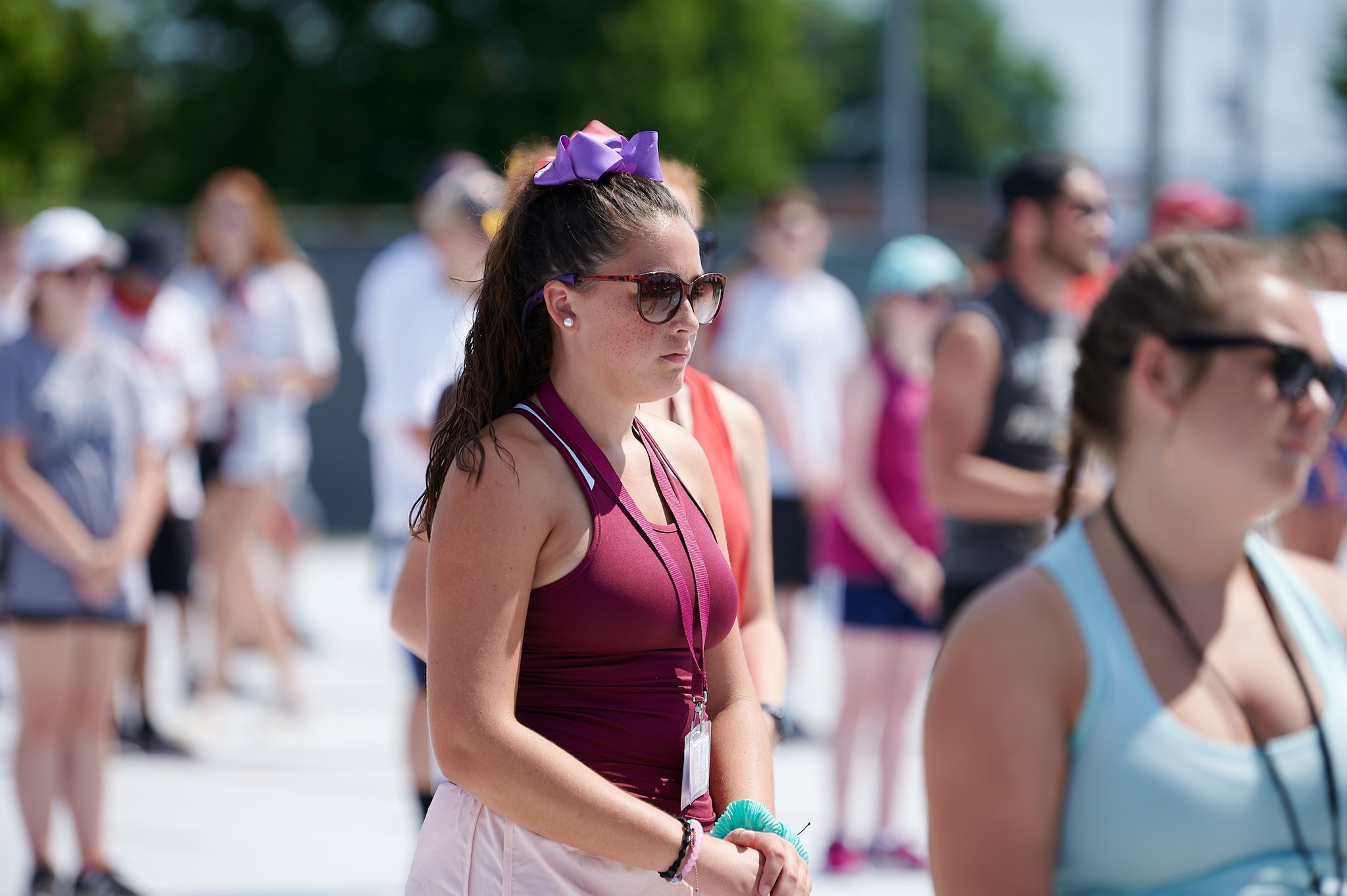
[[109, 718, 186, 755], [864, 843, 927, 869], [828, 840, 853, 870], [75, 872, 136, 896], [31, 868, 54, 896]]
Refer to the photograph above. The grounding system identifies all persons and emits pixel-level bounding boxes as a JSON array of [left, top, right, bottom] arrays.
[[353, 134, 1347, 867], [401, 119, 813, 896], [922, 234, 1347, 896], [0, 168, 340, 896]]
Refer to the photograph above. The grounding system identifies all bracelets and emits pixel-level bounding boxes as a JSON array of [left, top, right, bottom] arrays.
[[658, 816, 703, 891], [708, 800, 810, 865]]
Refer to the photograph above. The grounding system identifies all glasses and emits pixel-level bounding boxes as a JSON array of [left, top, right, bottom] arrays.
[[534, 271, 727, 326], [1123, 333, 1347, 427]]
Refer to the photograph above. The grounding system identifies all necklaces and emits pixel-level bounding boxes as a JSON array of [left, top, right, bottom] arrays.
[[1106, 489, 1345, 896]]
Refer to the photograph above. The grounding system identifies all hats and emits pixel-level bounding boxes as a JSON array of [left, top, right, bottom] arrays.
[[25, 208, 126, 272], [867, 235, 975, 296], [128, 231, 170, 278]]
